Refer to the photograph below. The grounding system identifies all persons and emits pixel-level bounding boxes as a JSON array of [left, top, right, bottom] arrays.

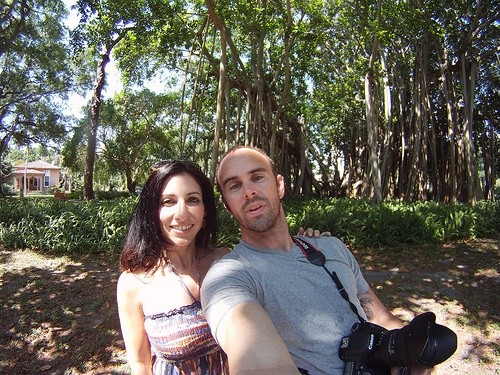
[[199, 146, 436, 375], [115, 159, 229, 375]]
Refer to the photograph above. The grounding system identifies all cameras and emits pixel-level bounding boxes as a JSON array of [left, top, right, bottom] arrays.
[[338, 312, 457, 375]]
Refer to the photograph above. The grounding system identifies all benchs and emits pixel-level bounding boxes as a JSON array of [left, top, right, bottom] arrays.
[[54, 191, 68, 200]]
